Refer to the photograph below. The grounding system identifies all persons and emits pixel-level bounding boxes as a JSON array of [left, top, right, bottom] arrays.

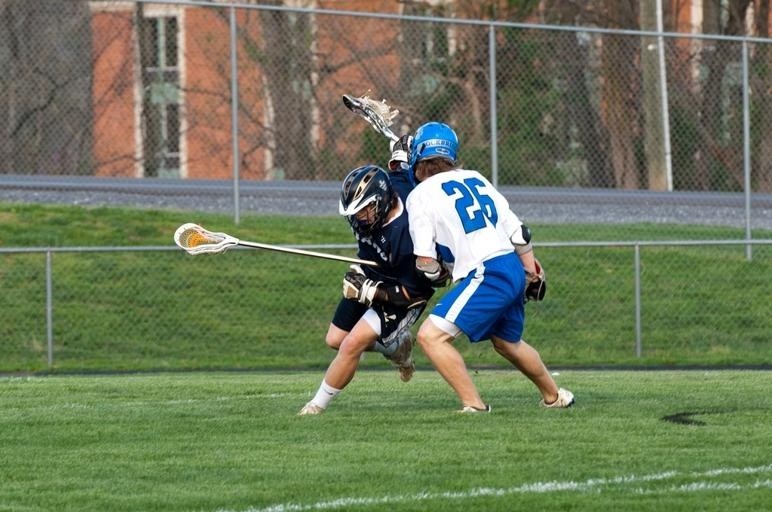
[[295, 135, 450, 416], [404, 121, 574, 415]]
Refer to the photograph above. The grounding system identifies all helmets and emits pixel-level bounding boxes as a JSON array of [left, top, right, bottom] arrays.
[[339, 165, 394, 235], [408, 122, 459, 187]]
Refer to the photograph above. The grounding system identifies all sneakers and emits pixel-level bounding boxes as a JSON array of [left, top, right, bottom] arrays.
[[457, 405, 492, 412], [298, 402, 323, 415], [385, 331, 415, 383], [540, 389, 574, 408]]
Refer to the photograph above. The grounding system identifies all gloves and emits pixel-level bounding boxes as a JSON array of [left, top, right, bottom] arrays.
[[343, 264, 385, 309], [525, 258, 546, 302], [388, 135, 413, 172]]
